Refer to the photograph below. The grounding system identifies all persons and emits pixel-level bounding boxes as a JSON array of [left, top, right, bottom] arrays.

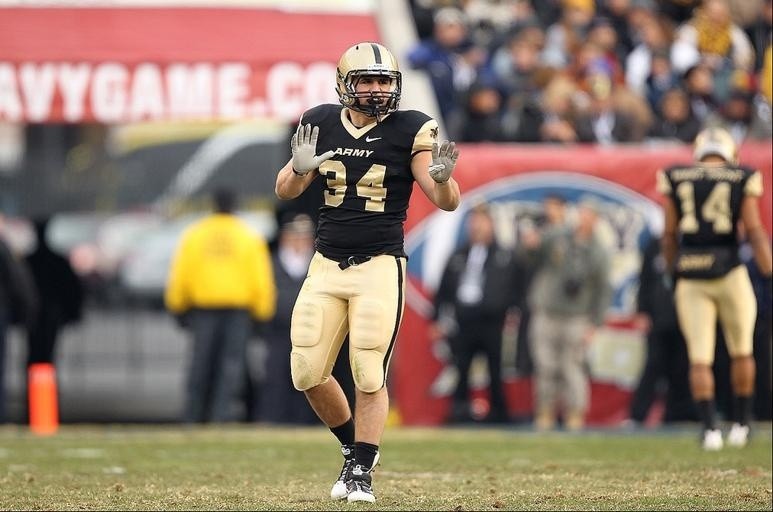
[[659, 126, 769, 453], [426, 195, 773, 430], [275, 41, 461, 505], [1, 188, 317, 423], [403, 1, 772, 139]]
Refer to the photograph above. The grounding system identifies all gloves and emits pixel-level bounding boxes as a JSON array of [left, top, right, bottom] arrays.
[[291, 123, 334, 176], [429, 140, 459, 183]]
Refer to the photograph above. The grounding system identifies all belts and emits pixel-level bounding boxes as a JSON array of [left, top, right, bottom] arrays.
[[324, 254, 371, 270]]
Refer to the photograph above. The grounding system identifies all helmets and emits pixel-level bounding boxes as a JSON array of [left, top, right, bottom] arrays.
[[694, 126, 737, 165], [335, 43, 401, 117]]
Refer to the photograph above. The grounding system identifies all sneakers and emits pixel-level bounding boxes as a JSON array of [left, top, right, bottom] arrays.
[[703, 422, 750, 450], [330, 445, 375, 502]]
